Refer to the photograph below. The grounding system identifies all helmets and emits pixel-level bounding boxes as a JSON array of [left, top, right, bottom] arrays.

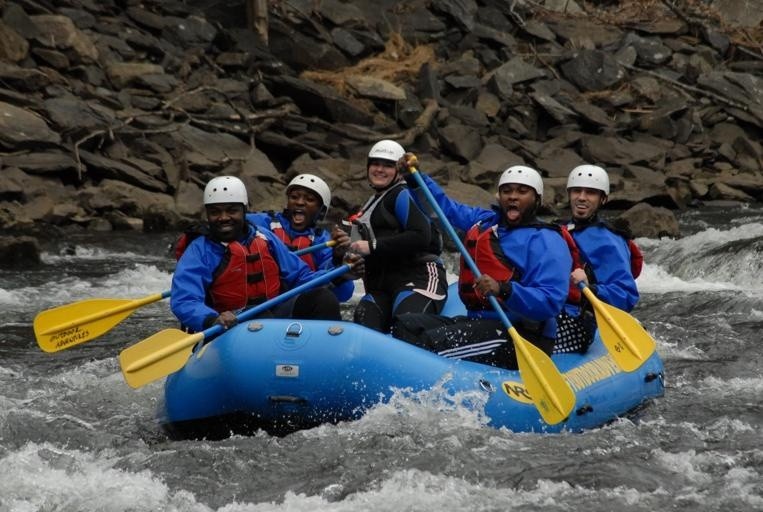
[[203, 175, 249, 206], [285, 173, 332, 221], [566, 164, 610, 197], [368, 139, 407, 163], [498, 165, 544, 196]]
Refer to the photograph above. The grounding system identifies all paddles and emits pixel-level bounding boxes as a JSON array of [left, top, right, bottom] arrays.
[[571, 271, 656, 372], [120, 260, 352, 388], [406, 157, 575, 425], [34, 240, 341, 353]]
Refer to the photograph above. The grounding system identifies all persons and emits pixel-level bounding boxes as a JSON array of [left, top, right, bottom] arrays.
[[170, 176, 365, 343], [552, 164, 639, 354], [245, 174, 355, 302], [350, 140, 449, 333], [389, 152, 573, 370]]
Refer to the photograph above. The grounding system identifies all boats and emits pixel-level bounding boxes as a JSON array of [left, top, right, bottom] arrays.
[[164, 282, 664, 433]]
